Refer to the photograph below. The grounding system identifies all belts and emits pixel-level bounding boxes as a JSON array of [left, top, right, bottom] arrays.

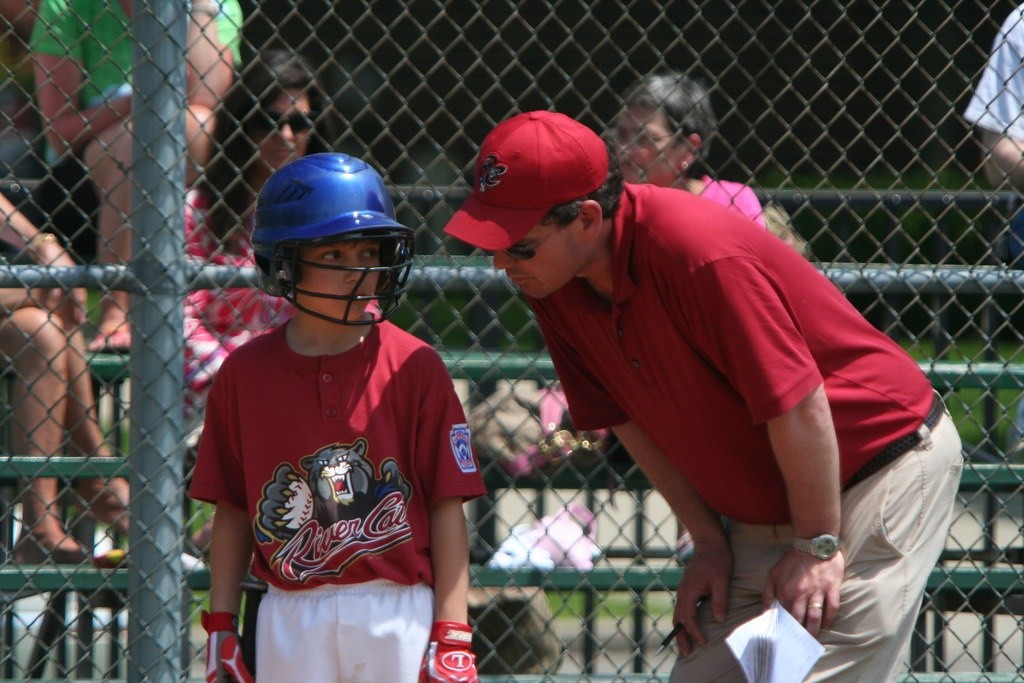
[[841, 391, 947, 495]]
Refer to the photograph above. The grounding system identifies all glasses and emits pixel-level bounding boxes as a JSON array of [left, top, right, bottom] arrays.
[[482, 243, 536, 260], [244, 107, 321, 136]]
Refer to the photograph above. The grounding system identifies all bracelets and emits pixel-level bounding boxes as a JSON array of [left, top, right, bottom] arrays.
[[27, 233, 56, 259]]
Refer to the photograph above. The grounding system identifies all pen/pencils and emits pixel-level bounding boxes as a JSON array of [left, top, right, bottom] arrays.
[[655, 597, 706, 657]]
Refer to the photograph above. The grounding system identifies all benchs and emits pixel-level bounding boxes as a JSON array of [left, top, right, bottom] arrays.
[[1, 344, 1022, 683]]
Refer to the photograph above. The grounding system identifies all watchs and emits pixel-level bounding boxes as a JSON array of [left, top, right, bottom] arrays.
[[788, 535, 842, 562]]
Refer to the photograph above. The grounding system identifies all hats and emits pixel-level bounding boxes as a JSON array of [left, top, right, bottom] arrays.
[[442, 109, 609, 250]]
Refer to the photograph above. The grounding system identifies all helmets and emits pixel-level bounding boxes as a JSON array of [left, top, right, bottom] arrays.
[[250, 152, 415, 327]]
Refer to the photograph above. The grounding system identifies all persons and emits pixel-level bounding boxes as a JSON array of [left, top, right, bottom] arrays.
[[960, 2, 1024, 266], [1, 1, 52, 176], [613, 69, 771, 233], [180, 49, 341, 549], [30, 0, 244, 359], [441, 111, 965, 682], [0, 192, 135, 562], [196, 154, 491, 682]]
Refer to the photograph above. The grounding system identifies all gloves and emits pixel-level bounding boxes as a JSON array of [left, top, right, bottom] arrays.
[[201, 610, 254, 683], [418, 621, 478, 683]]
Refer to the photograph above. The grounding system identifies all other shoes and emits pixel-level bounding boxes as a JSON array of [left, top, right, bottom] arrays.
[[88, 326, 131, 352], [21, 533, 89, 565], [83, 483, 131, 531]]
